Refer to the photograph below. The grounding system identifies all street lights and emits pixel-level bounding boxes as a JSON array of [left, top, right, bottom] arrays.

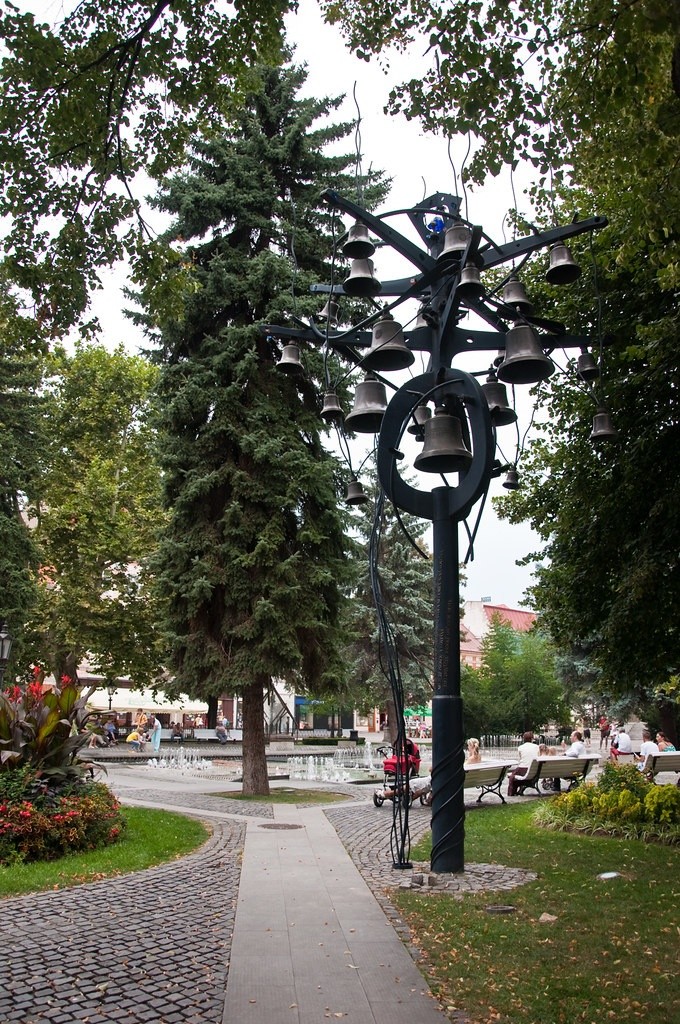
[[107, 684, 116, 709], [0, 620, 18, 692]]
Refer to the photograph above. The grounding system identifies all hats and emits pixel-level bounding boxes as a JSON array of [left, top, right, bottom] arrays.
[[523, 732, 533, 741]]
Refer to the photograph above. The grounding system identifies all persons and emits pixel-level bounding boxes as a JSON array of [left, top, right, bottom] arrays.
[[581, 715, 591, 747], [375, 767, 433, 803], [380, 716, 432, 739], [183, 714, 244, 744], [607, 728, 633, 762], [161, 720, 185, 744], [598, 715, 610, 753], [633, 731, 676, 782], [81, 714, 119, 749], [506, 731, 586, 791], [126, 709, 161, 752], [464, 738, 481, 764], [300, 720, 311, 729], [613, 718, 624, 732], [544, 721, 560, 737]]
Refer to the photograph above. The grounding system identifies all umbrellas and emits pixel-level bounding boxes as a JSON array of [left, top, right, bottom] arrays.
[[403, 703, 432, 717]]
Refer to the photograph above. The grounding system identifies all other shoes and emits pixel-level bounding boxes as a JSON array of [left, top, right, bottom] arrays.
[[94, 746, 98, 748], [551, 787, 561, 791], [374, 788, 383, 798], [89, 746, 94, 748]]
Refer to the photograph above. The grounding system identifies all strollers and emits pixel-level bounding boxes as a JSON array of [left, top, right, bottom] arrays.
[[373, 739, 422, 807], [536, 734, 579, 791]]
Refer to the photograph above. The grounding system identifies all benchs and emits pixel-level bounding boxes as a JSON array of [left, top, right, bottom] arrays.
[[640, 750, 680, 781], [149, 729, 181, 744], [420, 761, 520, 806], [513, 753, 602, 797], [193, 728, 244, 744]]
